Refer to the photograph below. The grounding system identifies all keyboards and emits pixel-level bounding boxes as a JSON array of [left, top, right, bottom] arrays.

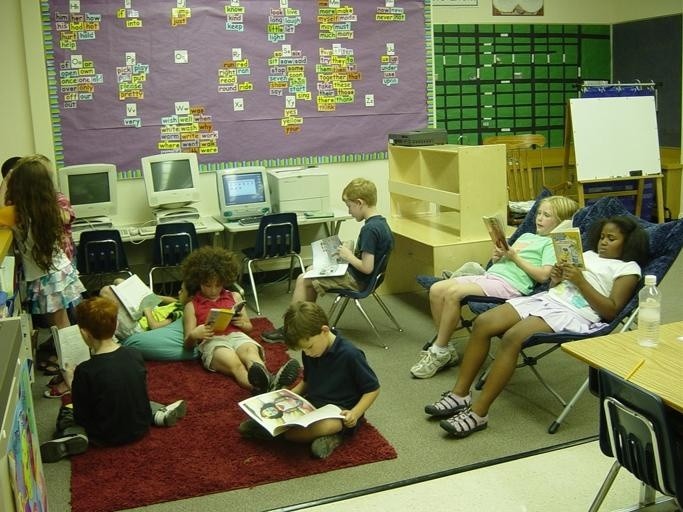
[[138, 220, 208, 235], [239, 217, 263, 226], [72, 228, 130, 242]]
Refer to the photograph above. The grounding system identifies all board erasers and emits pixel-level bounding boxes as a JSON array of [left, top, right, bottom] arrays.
[[629, 170, 643, 177]]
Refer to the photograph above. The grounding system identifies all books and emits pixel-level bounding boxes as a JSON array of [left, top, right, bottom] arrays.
[[482, 212, 510, 251], [302, 235, 355, 278], [206, 300, 247, 332], [108, 274, 163, 321], [238, 388, 346, 437], [550, 219, 586, 271]]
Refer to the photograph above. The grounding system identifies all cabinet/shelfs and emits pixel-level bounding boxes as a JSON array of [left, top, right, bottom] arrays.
[[372, 143, 518, 330], [0, 227, 47, 512]]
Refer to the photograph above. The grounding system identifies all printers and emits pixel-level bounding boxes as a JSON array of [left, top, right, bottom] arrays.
[[266, 166, 330, 214]]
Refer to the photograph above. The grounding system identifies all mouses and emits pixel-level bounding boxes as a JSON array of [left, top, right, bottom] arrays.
[[129, 227, 138, 235]]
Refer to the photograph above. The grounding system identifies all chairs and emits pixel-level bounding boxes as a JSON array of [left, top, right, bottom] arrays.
[[78, 229, 133, 278], [146, 222, 200, 298], [239, 213, 306, 316], [586, 368, 683, 512], [482, 134, 573, 227], [326, 255, 403, 350]]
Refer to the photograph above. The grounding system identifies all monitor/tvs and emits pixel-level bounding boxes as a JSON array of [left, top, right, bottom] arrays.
[[140, 152, 202, 217], [58, 163, 117, 226], [215, 166, 272, 220]]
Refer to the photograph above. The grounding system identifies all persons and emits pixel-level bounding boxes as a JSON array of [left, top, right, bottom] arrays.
[[0, 156, 96, 400], [258, 178, 394, 344], [236, 300, 382, 459], [410, 195, 580, 379], [427, 215, 652, 440], [178, 246, 299, 395], [100, 274, 198, 338], [38, 295, 186, 462]]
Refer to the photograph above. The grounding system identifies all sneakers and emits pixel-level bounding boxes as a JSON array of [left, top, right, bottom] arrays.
[[40, 433, 89, 464], [439, 406, 487, 438], [409, 343, 459, 379], [153, 399, 185, 427], [310, 432, 343, 458], [260, 325, 290, 344], [424, 390, 472, 417], [237, 418, 277, 440], [247, 361, 270, 396], [268, 358, 300, 393]]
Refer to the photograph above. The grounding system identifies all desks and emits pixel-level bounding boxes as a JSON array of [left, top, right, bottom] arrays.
[[212, 209, 346, 308], [72, 216, 224, 247]]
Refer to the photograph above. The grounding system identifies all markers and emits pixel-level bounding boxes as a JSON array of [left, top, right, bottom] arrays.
[[648, 173, 660, 175]]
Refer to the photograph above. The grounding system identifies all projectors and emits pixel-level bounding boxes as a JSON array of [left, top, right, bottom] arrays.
[[387, 127, 449, 146]]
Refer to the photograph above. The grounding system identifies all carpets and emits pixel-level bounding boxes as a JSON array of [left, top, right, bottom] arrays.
[[61, 317, 397, 512]]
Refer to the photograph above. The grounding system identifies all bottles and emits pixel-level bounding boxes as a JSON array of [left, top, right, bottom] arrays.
[[638, 274, 662, 348]]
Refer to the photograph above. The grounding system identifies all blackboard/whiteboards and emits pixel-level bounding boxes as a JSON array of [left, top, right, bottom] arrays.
[[569, 96, 662, 183], [612, 13, 683, 150]]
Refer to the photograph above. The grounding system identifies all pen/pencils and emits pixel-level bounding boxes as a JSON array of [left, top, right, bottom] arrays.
[[624, 359, 645, 381]]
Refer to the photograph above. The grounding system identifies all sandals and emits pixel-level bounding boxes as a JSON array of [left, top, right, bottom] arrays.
[[48, 375, 64, 387], [43, 384, 71, 398], [38, 355, 60, 375]]
